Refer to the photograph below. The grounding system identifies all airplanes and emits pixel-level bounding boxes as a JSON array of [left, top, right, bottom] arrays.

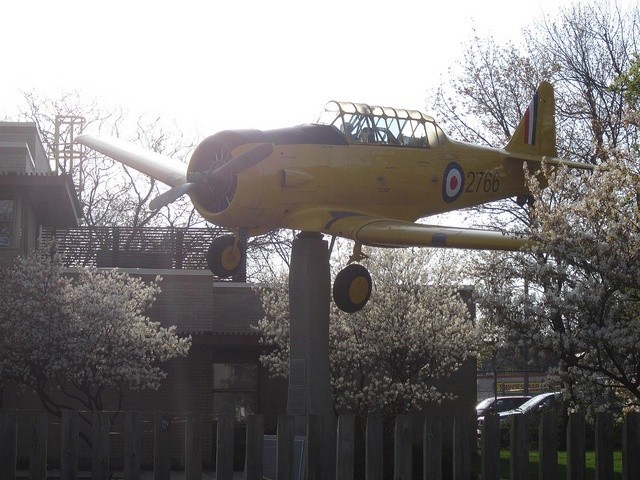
[[73, 81, 609, 310]]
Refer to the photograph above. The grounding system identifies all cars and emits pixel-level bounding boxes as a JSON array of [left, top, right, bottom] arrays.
[[476, 394, 531, 444], [496, 393, 604, 442]]
